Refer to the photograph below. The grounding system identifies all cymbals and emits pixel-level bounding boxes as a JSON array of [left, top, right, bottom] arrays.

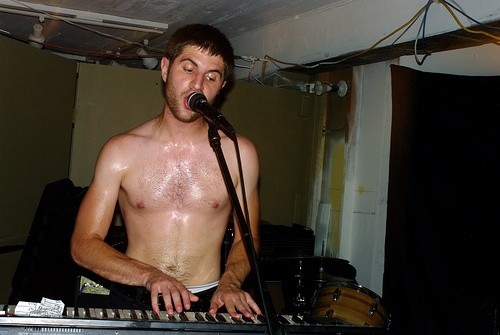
[[270, 256, 321, 260]]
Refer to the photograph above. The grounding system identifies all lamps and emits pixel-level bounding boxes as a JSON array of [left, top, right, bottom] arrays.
[[136, 45, 159, 70], [27, 23, 46, 49], [313, 79, 349, 97]]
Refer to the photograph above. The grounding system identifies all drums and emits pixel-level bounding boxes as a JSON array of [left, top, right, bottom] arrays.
[[303, 281, 392, 331]]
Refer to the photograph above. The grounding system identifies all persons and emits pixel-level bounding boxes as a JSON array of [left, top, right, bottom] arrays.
[[70, 23, 261, 318]]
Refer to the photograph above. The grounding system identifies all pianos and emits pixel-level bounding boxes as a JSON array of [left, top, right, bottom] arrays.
[[0, 302, 387, 335]]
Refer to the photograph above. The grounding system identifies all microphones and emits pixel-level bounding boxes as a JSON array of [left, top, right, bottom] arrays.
[[187, 92, 236, 137]]
